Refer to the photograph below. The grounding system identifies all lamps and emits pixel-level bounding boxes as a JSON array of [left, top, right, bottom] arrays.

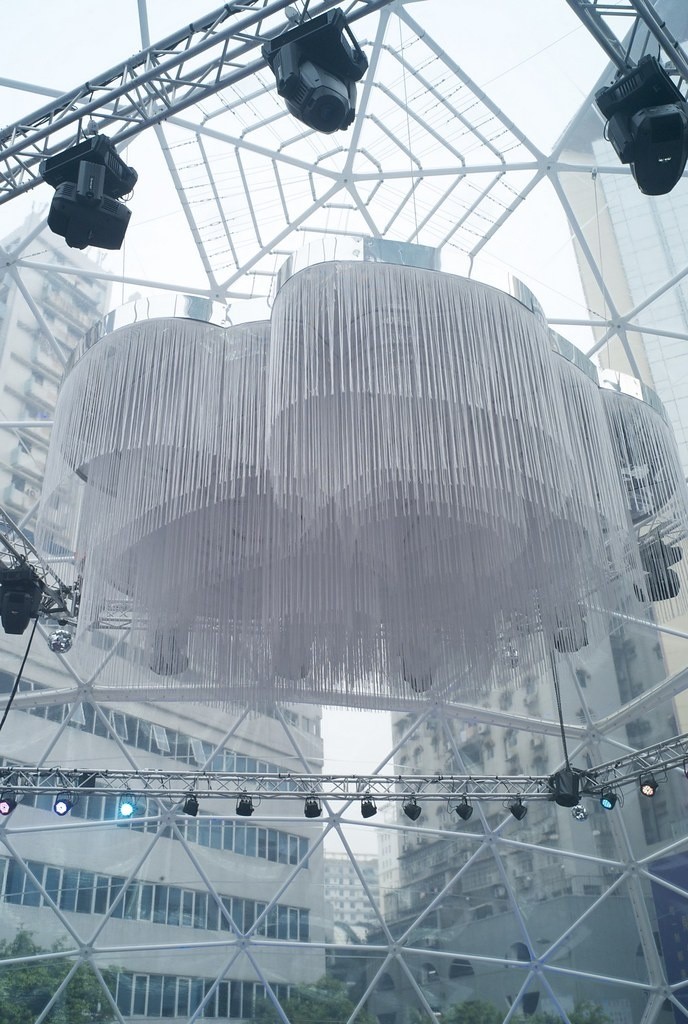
[[1, 771, 666, 817], [632, 538, 682, 603], [262, 8, 369, 134], [0, 568, 43, 635], [595, 54, 688, 195], [34, 134, 138, 251]]
